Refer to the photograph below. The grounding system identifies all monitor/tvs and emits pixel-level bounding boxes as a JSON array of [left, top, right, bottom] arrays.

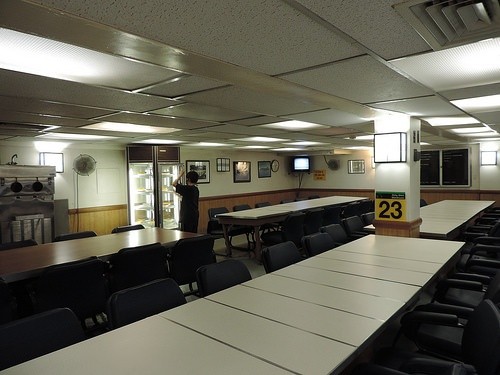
[[290, 156, 311, 173]]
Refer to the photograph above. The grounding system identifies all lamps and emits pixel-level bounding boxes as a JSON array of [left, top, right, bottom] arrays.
[[374, 132, 407, 163]]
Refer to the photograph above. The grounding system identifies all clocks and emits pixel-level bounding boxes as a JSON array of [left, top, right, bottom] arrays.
[[271, 159, 279, 172]]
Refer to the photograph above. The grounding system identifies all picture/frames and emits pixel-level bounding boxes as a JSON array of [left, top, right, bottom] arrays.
[[186, 160, 210, 184], [233, 161, 251, 182], [258, 160, 271, 177]]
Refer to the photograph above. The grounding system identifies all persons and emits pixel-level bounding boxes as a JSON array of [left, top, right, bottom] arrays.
[[172, 170, 199, 234]]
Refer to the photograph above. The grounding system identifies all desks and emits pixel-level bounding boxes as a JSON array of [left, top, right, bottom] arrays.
[[0, 228, 208, 292], [214, 195, 369, 260], [364, 199, 495, 238], [0, 234, 466, 375]]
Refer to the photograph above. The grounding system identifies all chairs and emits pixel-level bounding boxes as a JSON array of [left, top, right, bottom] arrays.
[[0, 196, 500, 375]]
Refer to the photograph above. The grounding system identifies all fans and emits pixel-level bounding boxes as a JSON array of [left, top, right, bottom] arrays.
[[72, 154, 97, 177]]
